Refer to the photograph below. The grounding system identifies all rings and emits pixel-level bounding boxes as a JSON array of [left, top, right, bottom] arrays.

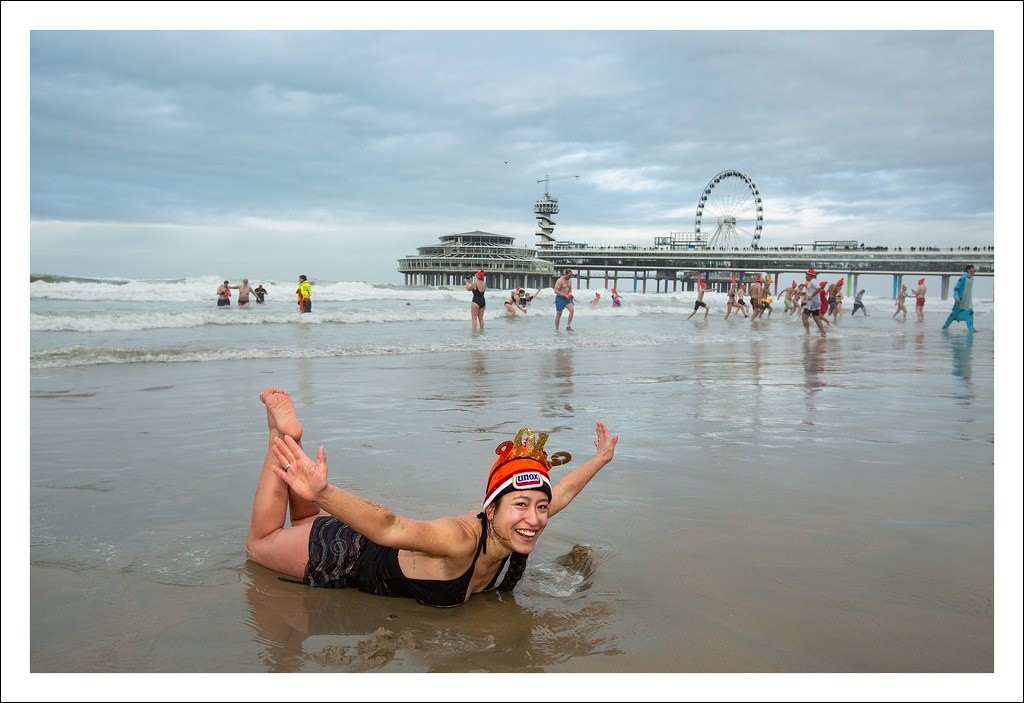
[[284, 463, 291, 470]]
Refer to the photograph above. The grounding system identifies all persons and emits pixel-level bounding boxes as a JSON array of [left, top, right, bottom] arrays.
[[912, 277, 927, 322], [798, 266, 827, 339], [295, 275, 313, 312], [245, 385, 619, 608], [463, 269, 487, 333], [723, 273, 846, 326], [687, 277, 710, 322], [553, 268, 576, 333], [851, 289, 870, 317], [504, 285, 541, 318], [253, 284, 268, 304], [570, 288, 624, 307], [216, 280, 232, 307], [221, 278, 261, 307], [891, 284, 916, 320], [942, 264, 981, 334]]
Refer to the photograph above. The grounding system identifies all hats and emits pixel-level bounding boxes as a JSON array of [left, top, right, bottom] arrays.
[[518, 288, 525, 293], [567, 293, 573, 298], [612, 288, 616, 293], [700, 283, 706, 287], [731, 268, 844, 289], [477, 270, 484, 279], [596, 293, 600, 297], [480, 428, 572, 513], [901, 278, 925, 288]]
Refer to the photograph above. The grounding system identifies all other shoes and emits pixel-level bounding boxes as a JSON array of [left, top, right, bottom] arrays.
[[566, 327, 574, 331]]
